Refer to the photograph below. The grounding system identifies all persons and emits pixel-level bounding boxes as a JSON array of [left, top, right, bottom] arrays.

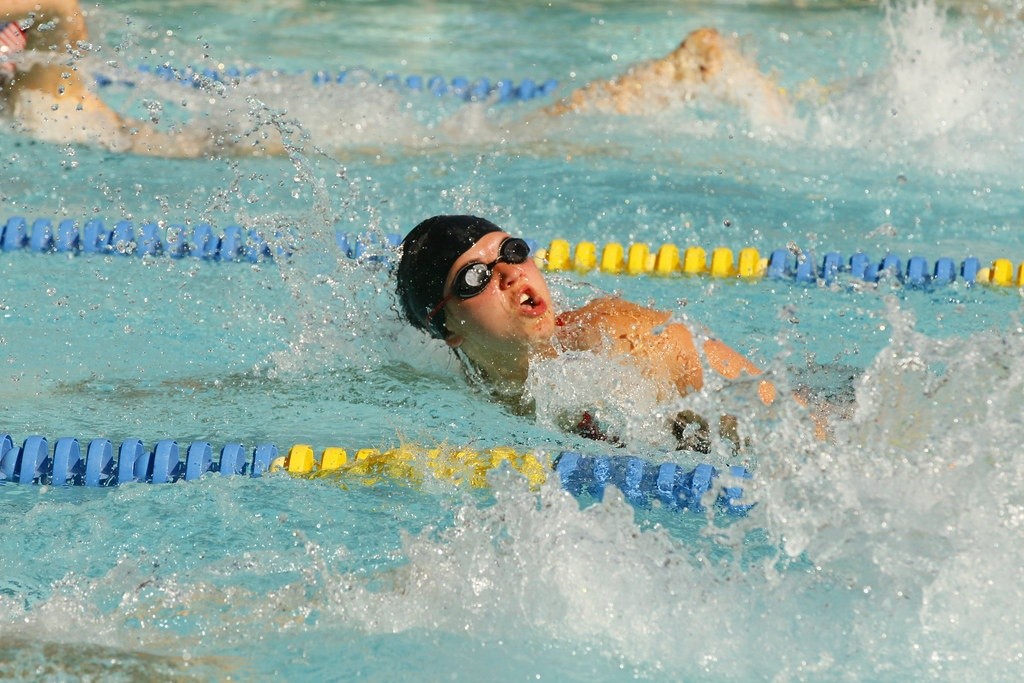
[[0, 0, 790, 159], [397, 214, 835, 465]]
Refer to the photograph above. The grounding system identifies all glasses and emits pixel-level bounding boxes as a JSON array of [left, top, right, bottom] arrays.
[[425, 237, 530, 327]]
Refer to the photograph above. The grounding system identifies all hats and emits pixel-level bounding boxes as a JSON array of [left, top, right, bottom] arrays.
[[395, 215, 506, 340]]
